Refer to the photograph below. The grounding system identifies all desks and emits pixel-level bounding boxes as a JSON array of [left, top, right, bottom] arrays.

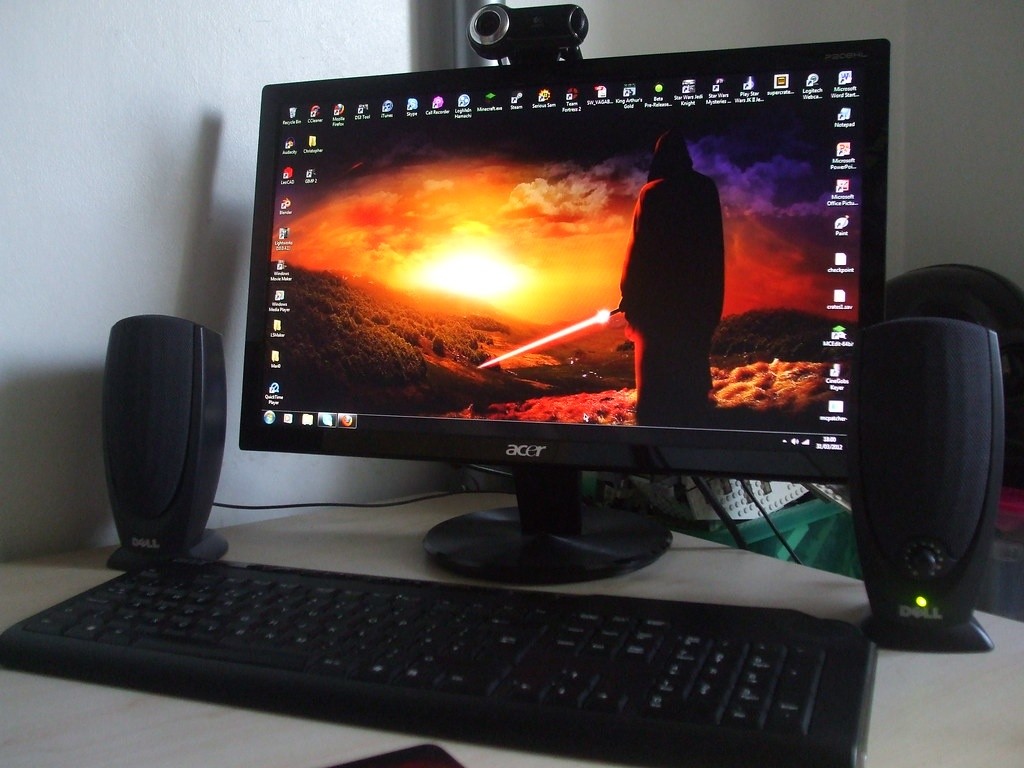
[[0, 487, 1024, 768]]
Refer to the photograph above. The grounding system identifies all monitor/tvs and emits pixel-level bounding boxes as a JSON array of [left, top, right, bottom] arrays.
[[239, 39, 893, 586]]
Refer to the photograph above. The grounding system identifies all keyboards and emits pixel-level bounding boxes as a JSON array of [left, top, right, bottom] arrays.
[[0, 562, 874, 768]]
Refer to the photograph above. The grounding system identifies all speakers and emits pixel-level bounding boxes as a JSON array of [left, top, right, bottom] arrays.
[[850, 316, 1006, 652], [101, 314, 229, 571]]
